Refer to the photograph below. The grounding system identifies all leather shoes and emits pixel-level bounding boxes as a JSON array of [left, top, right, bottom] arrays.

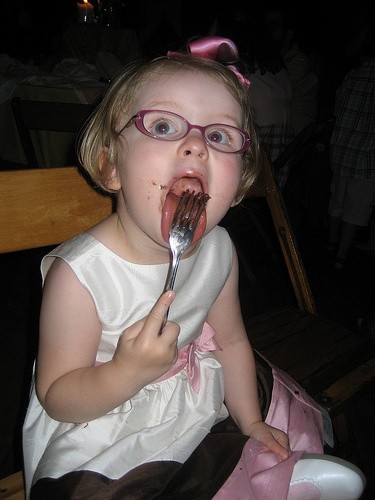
[[290, 453, 366, 500]]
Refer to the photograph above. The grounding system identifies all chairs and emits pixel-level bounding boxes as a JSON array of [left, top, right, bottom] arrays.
[[0, 150, 375, 500], [10, 97, 103, 169]]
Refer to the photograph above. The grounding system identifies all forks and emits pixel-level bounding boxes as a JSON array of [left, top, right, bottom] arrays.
[[158, 189, 211, 334]]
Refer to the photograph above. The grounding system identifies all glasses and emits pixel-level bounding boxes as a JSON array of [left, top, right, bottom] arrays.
[[116, 110, 252, 154]]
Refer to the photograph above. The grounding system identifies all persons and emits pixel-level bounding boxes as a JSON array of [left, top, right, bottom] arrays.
[[38, 0, 319, 204], [21, 36, 366, 500], [325, 31, 374, 274]]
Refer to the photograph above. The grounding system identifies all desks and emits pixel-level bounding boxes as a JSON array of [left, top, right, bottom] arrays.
[[0, 81, 107, 161]]
